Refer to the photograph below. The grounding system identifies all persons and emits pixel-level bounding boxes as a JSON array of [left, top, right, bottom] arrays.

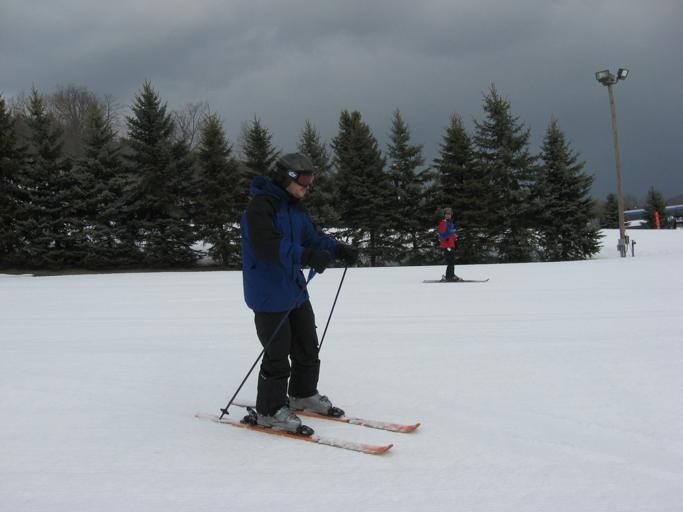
[[437, 206, 459, 282], [238, 150, 358, 432]]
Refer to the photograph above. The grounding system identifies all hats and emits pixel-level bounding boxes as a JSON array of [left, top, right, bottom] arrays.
[[272, 152, 314, 190], [439, 206, 453, 218]]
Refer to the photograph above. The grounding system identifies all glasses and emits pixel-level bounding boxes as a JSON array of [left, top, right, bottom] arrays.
[[277, 162, 314, 187]]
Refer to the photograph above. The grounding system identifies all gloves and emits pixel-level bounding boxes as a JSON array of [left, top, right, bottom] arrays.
[[335, 243, 358, 266], [303, 247, 332, 274]]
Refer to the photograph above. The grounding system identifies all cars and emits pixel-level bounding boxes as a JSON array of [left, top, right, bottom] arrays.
[[624, 205, 683, 228]]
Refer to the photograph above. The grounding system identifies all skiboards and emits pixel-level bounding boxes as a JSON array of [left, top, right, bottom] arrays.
[[422, 278, 489, 283], [194, 399, 419, 453]]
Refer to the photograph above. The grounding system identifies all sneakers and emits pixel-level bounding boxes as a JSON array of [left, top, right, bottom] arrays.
[[288, 392, 332, 411], [256, 405, 301, 426]]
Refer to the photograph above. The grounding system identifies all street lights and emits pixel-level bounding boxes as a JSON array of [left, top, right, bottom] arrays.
[[595, 68, 629, 257]]
